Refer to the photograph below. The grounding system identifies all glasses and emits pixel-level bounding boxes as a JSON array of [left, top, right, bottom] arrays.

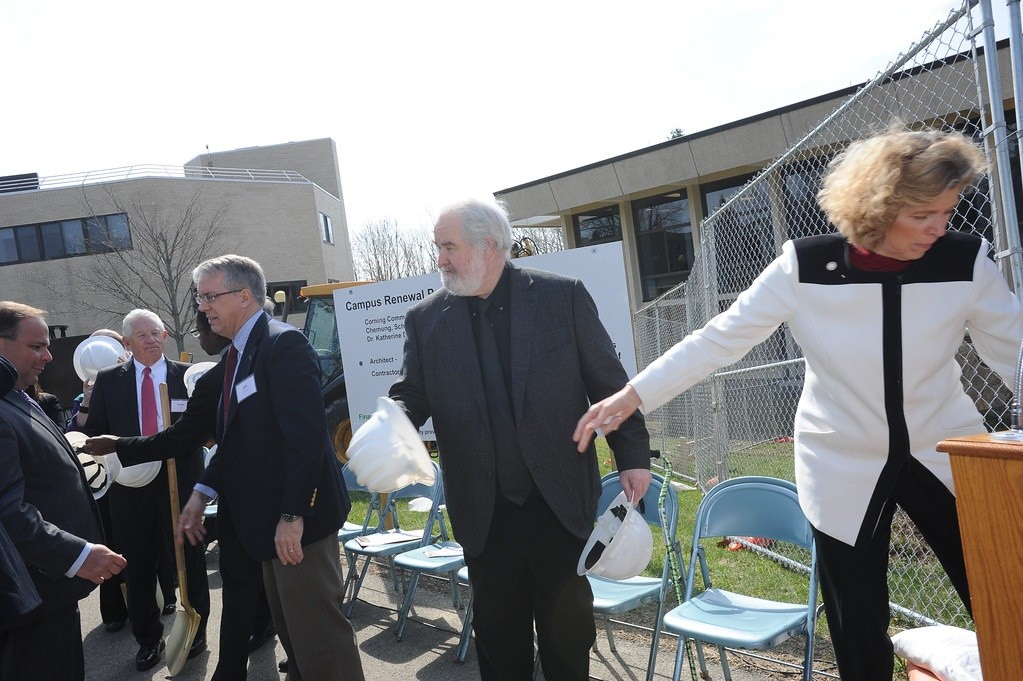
[[191, 329, 210, 338], [196, 290, 241, 304]]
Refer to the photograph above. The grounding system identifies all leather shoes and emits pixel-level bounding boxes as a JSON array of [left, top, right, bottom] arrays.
[[187, 633, 206, 660], [161, 604, 176, 615], [136, 637, 165, 670]]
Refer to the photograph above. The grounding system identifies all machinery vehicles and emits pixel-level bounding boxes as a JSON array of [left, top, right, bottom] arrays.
[[296, 236, 541, 468]]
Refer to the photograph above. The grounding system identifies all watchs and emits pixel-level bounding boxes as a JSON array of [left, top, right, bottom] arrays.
[[78, 404, 89, 414], [282, 514, 298, 522]]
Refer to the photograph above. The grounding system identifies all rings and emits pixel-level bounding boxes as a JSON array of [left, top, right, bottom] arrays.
[[289, 549, 294, 553]]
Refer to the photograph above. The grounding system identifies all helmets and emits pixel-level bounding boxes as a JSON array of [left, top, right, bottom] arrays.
[[581, 488, 653, 580], [73, 335, 126, 385], [347, 396, 435, 494], [184, 362, 220, 398], [65, 431, 121, 500], [114, 449, 162, 488]]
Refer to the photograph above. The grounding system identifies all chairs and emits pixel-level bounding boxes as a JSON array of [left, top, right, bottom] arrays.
[[338, 459, 826, 681]]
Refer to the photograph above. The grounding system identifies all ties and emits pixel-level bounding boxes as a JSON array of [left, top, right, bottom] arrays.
[[221, 344, 239, 445], [478, 300, 533, 506], [142, 368, 158, 436], [19, 389, 46, 416]]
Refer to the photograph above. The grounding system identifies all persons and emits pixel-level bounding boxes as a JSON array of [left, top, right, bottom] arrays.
[[571, 116, 1023, 681], [0, 253, 366, 680], [388, 191, 661, 681]]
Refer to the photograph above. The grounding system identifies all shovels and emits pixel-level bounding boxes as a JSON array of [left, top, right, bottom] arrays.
[[158, 383, 201, 676]]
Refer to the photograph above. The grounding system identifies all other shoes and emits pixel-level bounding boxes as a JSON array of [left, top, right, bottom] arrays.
[[246, 623, 277, 651], [279, 658, 289, 671], [106, 609, 128, 632]]
